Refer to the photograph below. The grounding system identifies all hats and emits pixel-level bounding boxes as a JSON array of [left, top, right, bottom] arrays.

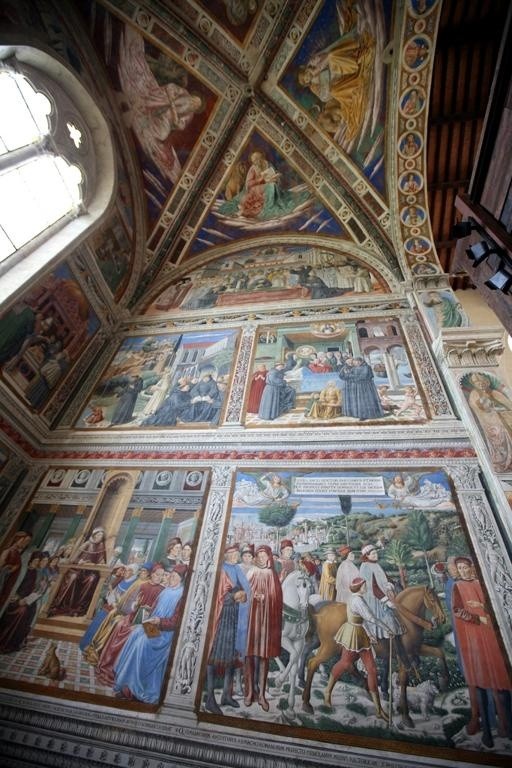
[[240, 544, 254, 558], [225, 543, 239, 553], [14, 531, 73, 568], [323, 546, 337, 554], [91, 526, 104, 537], [280, 540, 293, 553], [454, 557, 472, 567], [351, 577, 365, 586], [361, 544, 377, 556], [114, 538, 193, 582], [301, 559, 315, 575], [336, 546, 352, 558]]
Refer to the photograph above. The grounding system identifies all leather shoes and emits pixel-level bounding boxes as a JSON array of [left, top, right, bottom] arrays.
[[466, 714, 480, 736], [496, 720, 507, 737]]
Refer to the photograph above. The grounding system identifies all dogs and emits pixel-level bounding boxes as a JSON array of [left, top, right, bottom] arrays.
[[390, 671, 441, 721], [37, 642, 66, 681]]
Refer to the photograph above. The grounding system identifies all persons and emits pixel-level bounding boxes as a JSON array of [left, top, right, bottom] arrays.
[[152, 247, 380, 315], [1, 280, 87, 411], [397, 1, 449, 292], [421, 289, 464, 335], [288, 21, 379, 143], [209, 149, 315, 221], [113, 19, 210, 187], [467, 369, 512, 478]]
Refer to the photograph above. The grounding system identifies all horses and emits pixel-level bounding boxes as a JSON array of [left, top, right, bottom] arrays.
[[273, 569, 319, 718], [301, 584, 447, 729]]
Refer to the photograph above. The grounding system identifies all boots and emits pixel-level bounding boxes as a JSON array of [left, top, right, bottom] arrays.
[[370, 692, 389, 723], [475, 688, 494, 747], [205, 656, 270, 716], [322, 672, 337, 708], [498, 689, 511, 741]]
